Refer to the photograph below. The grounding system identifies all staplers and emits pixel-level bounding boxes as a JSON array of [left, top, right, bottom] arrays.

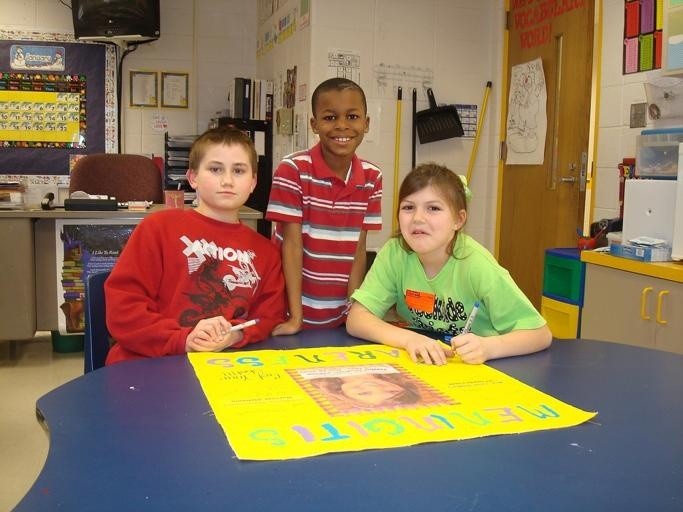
[[41, 193, 55, 210]]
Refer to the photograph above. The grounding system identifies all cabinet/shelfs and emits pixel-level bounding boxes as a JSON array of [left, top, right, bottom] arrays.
[[205, 117, 275, 243], [577, 251, 683, 354]]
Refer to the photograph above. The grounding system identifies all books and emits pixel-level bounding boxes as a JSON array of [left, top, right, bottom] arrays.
[[228, 77, 275, 120], [165, 132, 203, 184]]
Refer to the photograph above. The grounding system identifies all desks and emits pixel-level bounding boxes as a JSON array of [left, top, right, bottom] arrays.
[[1, 201, 264, 348], [10, 333, 683, 510]]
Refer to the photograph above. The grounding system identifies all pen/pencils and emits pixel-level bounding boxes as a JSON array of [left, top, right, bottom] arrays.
[[222, 318, 260, 335], [462, 301, 480, 335]]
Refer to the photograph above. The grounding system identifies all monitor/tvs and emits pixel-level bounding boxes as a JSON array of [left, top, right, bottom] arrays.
[[71, 0, 162, 42]]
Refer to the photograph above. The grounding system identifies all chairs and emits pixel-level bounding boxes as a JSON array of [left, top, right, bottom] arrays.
[[67, 150, 161, 205]]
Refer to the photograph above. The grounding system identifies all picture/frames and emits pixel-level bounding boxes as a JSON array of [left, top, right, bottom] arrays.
[[127, 69, 158, 106], [159, 71, 189, 110]]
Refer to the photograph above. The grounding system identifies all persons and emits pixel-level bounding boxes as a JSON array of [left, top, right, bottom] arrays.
[[311, 372, 419, 411], [346, 163, 553, 364], [102, 124, 287, 366], [265, 77, 383, 336]]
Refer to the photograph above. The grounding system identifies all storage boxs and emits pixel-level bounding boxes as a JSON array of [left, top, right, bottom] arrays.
[[538, 292, 584, 342], [539, 246, 588, 305], [634, 126, 683, 180]]
